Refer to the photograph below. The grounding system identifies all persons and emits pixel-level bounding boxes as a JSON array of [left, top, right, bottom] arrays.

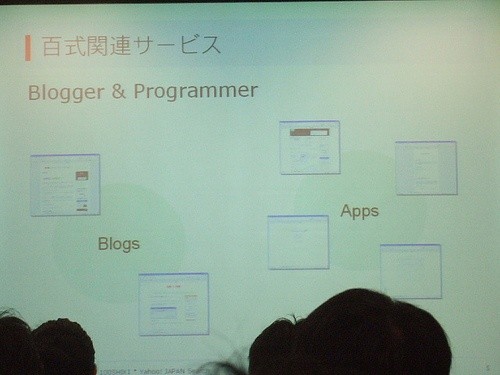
[[255, 289, 456, 374], [0, 309, 96, 374]]
